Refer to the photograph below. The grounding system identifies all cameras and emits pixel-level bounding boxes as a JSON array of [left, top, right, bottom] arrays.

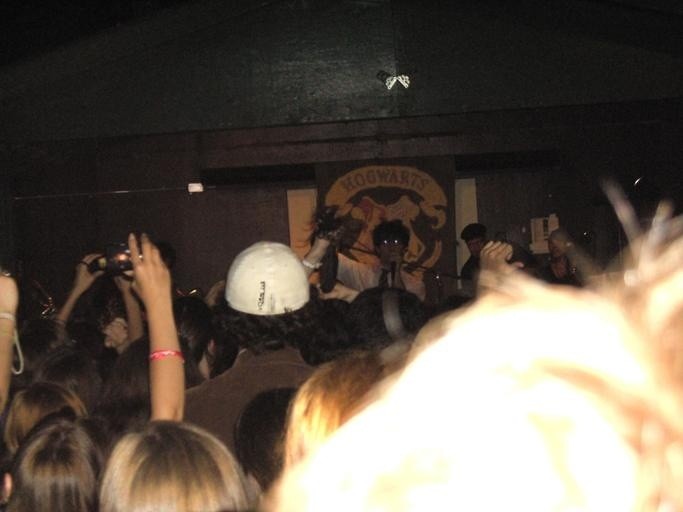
[[83, 228, 176, 284]]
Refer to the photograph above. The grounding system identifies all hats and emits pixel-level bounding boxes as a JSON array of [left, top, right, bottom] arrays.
[[226, 241, 311, 316]]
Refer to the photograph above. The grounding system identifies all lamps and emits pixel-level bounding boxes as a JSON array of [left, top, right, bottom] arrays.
[[186, 172, 206, 194], [376, 69, 411, 90]]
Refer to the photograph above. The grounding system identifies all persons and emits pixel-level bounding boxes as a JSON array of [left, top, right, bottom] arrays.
[[2, 200, 683, 511]]
